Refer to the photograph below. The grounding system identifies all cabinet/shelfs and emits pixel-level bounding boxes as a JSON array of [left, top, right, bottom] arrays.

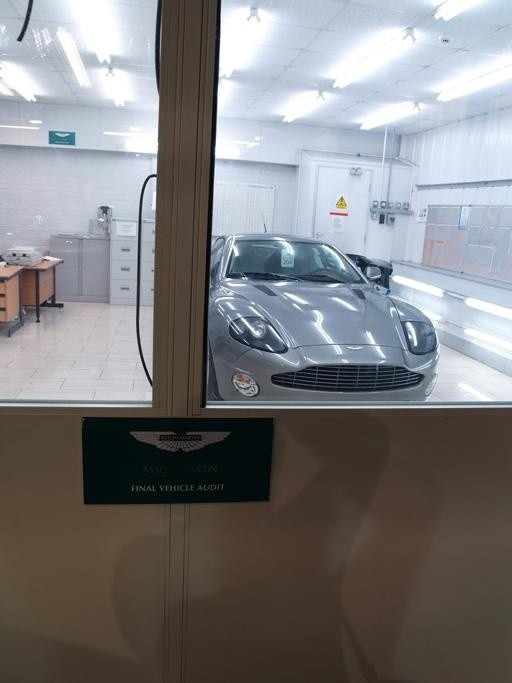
[[49, 217, 156, 308]]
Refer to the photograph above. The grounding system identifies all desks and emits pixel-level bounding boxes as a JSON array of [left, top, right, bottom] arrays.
[[0, 257, 64, 337]]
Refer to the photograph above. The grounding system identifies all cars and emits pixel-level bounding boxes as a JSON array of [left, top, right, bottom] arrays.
[[206, 232, 439, 401]]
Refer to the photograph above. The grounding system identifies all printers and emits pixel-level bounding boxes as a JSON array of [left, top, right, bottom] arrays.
[[6, 247, 43, 267]]
